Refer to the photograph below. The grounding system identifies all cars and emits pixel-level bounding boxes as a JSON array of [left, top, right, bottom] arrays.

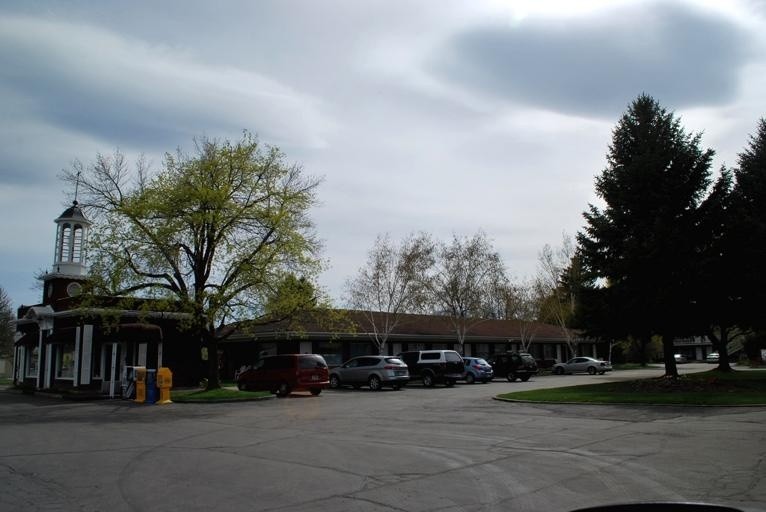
[[461, 356, 494, 385], [673, 353, 688, 365], [552, 356, 613, 376], [706, 352, 719, 362]]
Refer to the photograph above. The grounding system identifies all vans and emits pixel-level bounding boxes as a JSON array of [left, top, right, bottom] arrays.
[[392, 349, 464, 387]]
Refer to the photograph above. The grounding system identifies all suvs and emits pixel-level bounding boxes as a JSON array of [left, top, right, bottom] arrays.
[[328, 356, 410, 392], [238, 353, 330, 397], [485, 353, 541, 383]]
